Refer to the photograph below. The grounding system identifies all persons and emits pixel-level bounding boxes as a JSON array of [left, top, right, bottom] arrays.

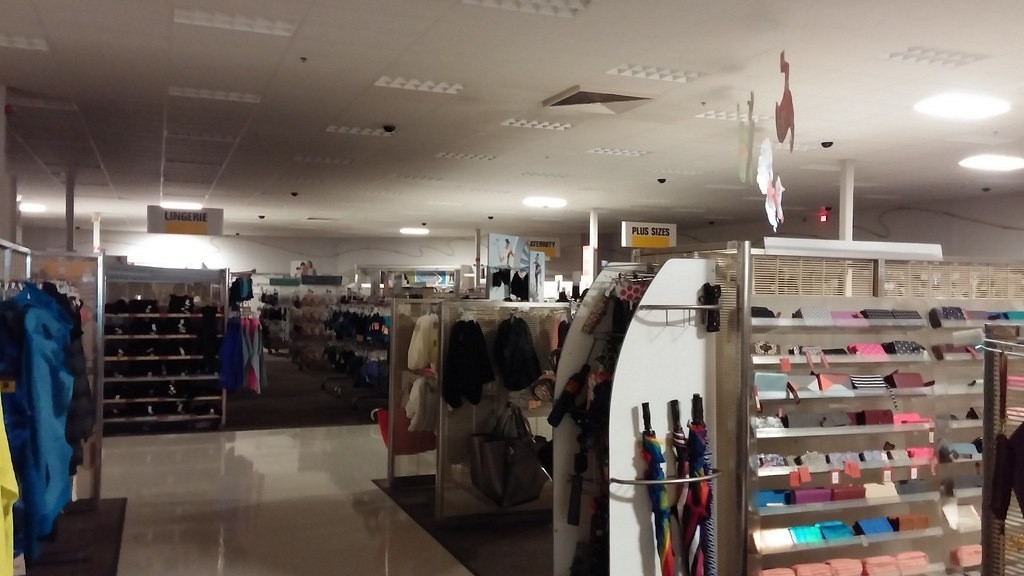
[[296, 260, 312, 276], [498, 239, 513, 265], [534, 253, 543, 294]]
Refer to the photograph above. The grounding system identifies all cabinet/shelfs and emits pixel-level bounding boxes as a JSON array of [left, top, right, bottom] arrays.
[[101, 264, 232, 430], [743, 294, 1024, 576]]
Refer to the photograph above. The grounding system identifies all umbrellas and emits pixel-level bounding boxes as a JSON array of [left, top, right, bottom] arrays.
[[642, 402, 675, 576], [547, 281, 637, 539], [671, 393, 716, 576]]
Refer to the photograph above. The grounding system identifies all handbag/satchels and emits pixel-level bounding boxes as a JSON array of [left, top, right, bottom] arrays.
[[471, 401, 547, 506]]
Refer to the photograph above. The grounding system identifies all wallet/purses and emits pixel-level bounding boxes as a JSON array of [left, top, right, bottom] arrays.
[[750, 304, 984, 554]]
